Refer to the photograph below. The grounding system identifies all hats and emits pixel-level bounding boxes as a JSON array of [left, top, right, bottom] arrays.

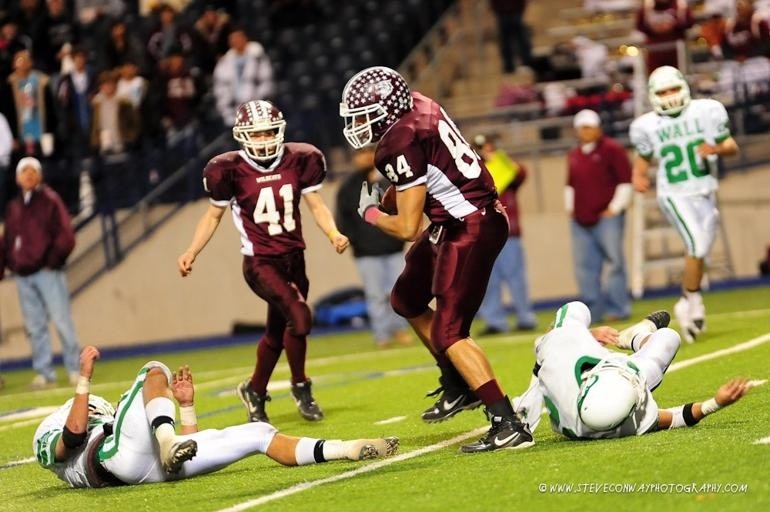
[[16, 156, 42, 173], [573, 109, 602, 129]]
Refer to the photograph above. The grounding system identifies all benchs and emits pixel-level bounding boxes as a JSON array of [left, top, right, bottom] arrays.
[[472, 1, 770, 167]]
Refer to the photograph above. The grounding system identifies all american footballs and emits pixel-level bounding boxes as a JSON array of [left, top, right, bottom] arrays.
[[381, 184, 398, 215]]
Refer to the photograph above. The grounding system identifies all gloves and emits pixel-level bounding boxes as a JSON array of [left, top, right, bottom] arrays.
[[357, 181, 385, 220]]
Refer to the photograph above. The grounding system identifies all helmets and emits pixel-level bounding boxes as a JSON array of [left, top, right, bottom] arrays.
[[577, 353, 647, 432], [232, 100, 287, 161], [648, 66, 691, 115], [340, 66, 413, 150], [63, 394, 116, 416]]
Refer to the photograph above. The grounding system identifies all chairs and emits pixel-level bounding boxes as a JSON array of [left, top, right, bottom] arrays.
[[1, 0, 454, 210]]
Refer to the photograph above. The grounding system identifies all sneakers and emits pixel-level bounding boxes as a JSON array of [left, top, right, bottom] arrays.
[[162, 440, 197, 481], [461, 416, 536, 453], [420, 376, 483, 423], [478, 322, 536, 336], [348, 436, 400, 461], [673, 289, 706, 345], [376, 329, 414, 350], [237, 377, 271, 422], [290, 379, 324, 421], [606, 311, 670, 349], [33, 372, 79, 385]]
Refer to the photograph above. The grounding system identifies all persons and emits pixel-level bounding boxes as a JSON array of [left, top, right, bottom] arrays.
[[490, 0, 770, 140], [176, 99, 350, 422], [33, 345, 400, 489], [0, 0, 275, 226], [563, 107, 634, 324], [338, 65, 536, 454], [0, 157, 81, 389], [471, 134, 536, 337], [511, 300, 751, 440], [335, 146, 413, 349], [628, 64, 740, 345]]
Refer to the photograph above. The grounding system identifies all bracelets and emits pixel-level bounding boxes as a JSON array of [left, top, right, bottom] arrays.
[[179, 405, 198, 426], [364, 206, 383, 225], [76, 376, 90, 395], [701, 398, 722, 416], [327, 228, 340, 242]]
